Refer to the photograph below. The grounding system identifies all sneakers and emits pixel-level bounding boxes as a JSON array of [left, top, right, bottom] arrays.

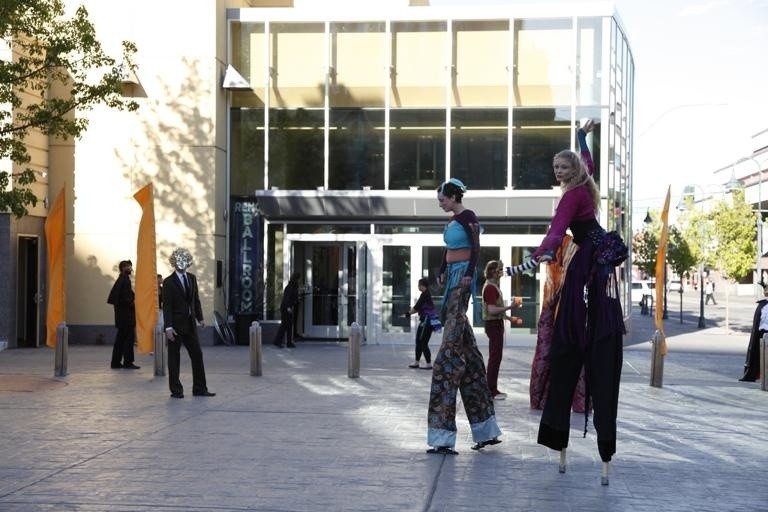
[[494, 393, 507, 399]]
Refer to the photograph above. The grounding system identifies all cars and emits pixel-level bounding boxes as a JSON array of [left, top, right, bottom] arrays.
[[666, 278, 682, 291]]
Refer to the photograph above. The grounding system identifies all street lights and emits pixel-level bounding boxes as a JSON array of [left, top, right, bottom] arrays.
[[725, 155, 763, 300]]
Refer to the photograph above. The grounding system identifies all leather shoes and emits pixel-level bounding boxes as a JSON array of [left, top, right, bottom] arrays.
[[421, 366, 432, 370], [111, 362, 123, 368], [170, 393, 184, 398], [123, 363, 140, 369], [409, 364, 419, 368], [192, 391, 216, 396]]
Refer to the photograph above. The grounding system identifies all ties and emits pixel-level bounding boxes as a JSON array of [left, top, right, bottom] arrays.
[[182, 273, 189, 295]]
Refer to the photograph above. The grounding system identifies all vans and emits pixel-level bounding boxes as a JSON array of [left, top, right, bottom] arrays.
[[631, 281, 657, 305]]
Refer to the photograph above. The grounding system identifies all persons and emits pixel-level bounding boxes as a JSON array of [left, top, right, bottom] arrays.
[[157, 274, 163, 309], [162, 247, 216, 398], [107, 260, 141, 370], [506, 229, 595, 416], [526, 120, 628, 486], [274, 273, 303, 348], [399, 277, 433, 369], [482, 259, 524, 401], [424, 177, 503, 456], [704, 277, 718, 306]]
